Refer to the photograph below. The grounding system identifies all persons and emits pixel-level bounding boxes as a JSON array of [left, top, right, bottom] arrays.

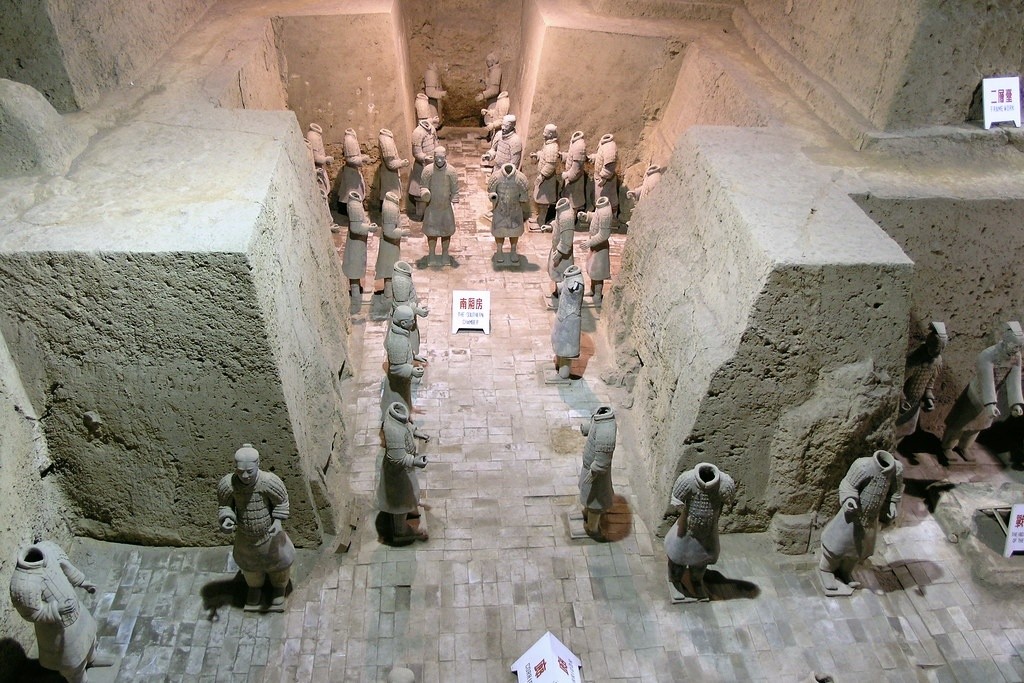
[[475, 52, 522, 185], [302, 123, 408, 296], [888, 322, 947, 459], [577, 196, 613, 304], [388, 305, 427, 412], [420, 146, 459, 265], [9, 542, 114, 683], [577, 406, 617, 535], [487, 162, 530, 263], [392, 260, 428, 362], [409, 63, 448, 220], [664, 462, 736, 600], [375, 191, 411, 303], [528, 123, 661, 231], [551, 265, 584, 378], [376, 402, 431, 536], [941, 321, 1024, 461], [218, 444, 295, 606], [818, 450, 904, 590], [541, 198, 574, 306]]
